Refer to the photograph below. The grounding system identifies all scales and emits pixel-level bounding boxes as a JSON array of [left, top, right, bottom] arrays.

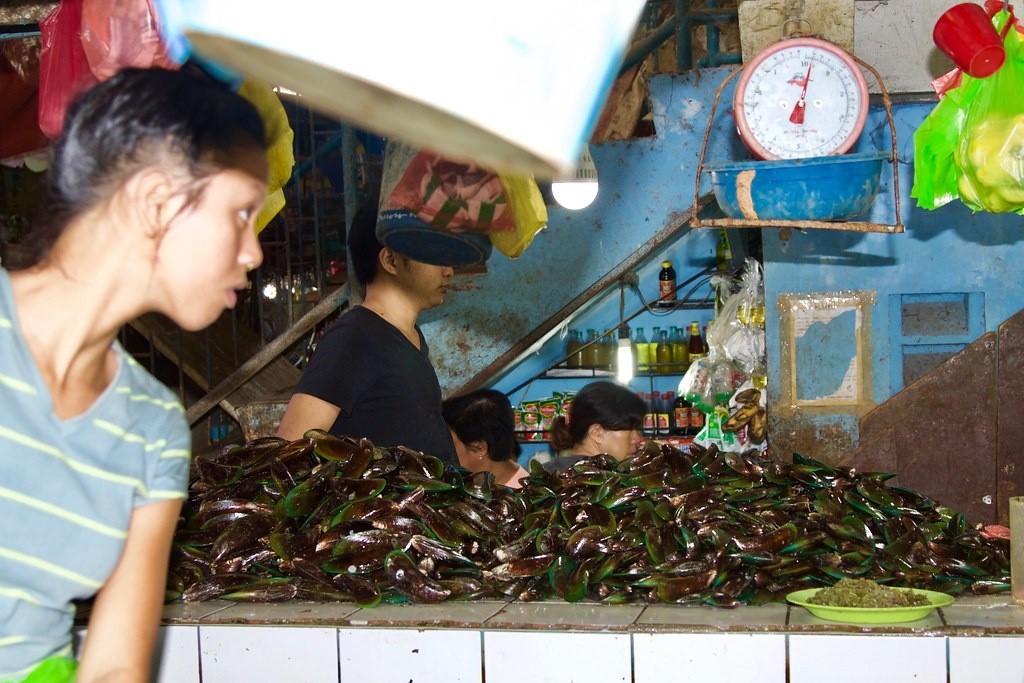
[[699, 18, 891, 223]]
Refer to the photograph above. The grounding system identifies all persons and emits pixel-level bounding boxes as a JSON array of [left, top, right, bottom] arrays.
[[548, 381, 648, 463], [275, 201, 461, 469], [0, 67, 266, 683], [442, 388, 532, 489]]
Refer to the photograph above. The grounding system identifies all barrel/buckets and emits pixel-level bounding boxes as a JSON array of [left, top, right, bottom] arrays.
[[376, 139, 494, 267]]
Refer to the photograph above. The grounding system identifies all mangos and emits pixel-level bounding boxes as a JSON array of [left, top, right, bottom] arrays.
[[952, 113, 1024, 213]]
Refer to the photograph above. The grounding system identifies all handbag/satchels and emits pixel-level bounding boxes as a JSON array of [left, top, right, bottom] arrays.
[[389, 147, 548, 259], [953, 9, 1024, 215], [36, 1, 242, 145]]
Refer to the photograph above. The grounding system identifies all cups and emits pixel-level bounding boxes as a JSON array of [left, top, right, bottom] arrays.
[[933, 2, 1015, 79], [1009, 496, 1024, 602]]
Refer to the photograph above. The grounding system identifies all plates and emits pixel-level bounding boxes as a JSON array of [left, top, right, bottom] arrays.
[[785, 587, 954, 623]]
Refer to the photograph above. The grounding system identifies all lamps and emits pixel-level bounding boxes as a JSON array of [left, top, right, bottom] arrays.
[[551, 144, 598, 209]]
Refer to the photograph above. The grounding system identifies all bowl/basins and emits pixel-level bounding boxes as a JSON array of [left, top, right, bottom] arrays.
[[702, 150, 892, 222]]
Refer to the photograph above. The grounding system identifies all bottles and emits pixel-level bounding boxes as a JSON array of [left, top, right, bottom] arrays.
[[635, 390, 704, 438], [565, 318, 714, 371], [659, 261, 677, 305]]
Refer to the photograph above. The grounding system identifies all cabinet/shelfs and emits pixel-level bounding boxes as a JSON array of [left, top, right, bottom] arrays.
[[514, 296, 715, 442]]
